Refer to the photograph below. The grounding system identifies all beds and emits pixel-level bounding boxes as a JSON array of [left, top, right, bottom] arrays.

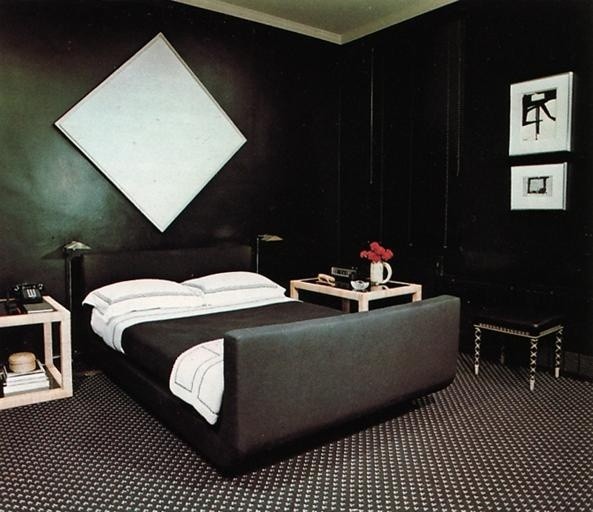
[[77, 244, 461, 469]]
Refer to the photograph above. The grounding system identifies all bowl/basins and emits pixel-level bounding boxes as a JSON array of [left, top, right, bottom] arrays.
[[350, 279, 370, 292]]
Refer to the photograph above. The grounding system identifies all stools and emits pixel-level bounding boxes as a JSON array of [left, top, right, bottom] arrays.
[[470, 303, 567, 391]]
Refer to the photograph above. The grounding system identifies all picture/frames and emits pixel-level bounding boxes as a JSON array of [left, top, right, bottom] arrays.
[[53, 32, 247, 232], [508, 72, 573, 211]]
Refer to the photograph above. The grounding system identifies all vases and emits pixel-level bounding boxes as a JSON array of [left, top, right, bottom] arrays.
[[369, 262, 392, 285]]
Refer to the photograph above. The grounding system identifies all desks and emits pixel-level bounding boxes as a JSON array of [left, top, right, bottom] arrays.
[[290, 275, 422, 313], [0, 296, 73, 412]]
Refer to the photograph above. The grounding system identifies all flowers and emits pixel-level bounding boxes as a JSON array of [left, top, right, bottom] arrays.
[[360, 242, 393, 263]]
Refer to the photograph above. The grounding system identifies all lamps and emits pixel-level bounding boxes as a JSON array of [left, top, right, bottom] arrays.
[[256, 235, 282, 272], [63, 241, 92, 312]]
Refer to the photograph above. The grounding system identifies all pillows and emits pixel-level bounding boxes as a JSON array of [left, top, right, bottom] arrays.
[[82, 270, 287, 325]]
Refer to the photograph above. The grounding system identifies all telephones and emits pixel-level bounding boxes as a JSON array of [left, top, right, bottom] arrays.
[[13, 282, 45, 303]]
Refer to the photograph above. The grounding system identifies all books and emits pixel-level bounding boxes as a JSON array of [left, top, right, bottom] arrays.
[[2, 356, 50, 394]]
